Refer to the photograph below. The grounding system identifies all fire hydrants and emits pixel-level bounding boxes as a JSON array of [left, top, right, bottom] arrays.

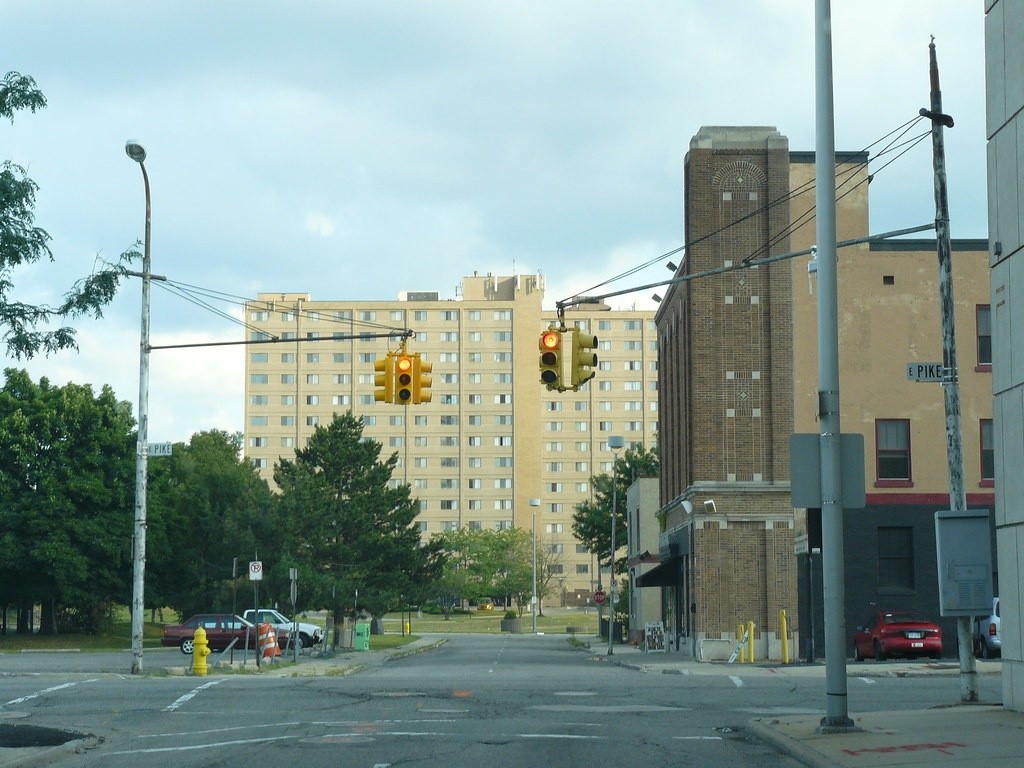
[[190, 627, 212, 676]]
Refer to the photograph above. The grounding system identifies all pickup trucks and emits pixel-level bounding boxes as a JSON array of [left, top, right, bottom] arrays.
[[205, 609, 325, 644]]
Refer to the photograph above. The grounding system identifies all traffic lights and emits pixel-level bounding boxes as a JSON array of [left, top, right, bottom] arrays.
[[372, 352, 395, 404], [415, 354, 434, 404], [537, 331, 565, 392], [395, 355, 414, 406], [562, 328, 601, 393]]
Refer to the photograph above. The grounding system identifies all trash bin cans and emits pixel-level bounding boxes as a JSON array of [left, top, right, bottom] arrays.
[[338, 627, 355, 648]]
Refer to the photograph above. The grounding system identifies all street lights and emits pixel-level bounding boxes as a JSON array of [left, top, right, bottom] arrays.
[[528, 498, 542, 633], [123, 139, 154, 675], [606, 435, 626, 658]]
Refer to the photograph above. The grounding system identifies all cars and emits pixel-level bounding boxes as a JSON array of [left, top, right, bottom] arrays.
[[971, 598, 1000, 660], [161, 613, 293, 655], [854, 602, 944, 662], [477, 597, 496, 610]]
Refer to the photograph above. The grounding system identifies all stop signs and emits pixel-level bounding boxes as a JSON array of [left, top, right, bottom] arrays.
[[594, 592, 606, 604]]
